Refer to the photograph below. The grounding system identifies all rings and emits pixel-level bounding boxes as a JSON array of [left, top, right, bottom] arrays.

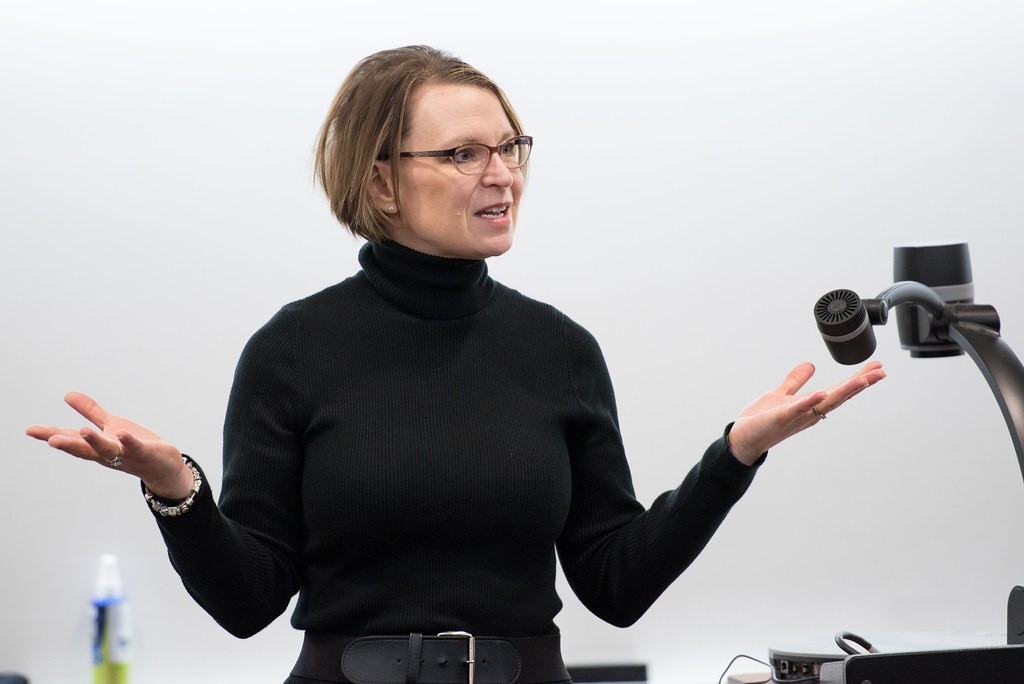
[[811, 403, 822, 420], [103, 445, 123, 467]]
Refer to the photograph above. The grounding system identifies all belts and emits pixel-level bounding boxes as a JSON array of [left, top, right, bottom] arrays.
[[288, 629, 574, 684]]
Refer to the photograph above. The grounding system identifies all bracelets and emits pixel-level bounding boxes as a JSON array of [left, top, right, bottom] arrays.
[[142, 456, 202, 516]]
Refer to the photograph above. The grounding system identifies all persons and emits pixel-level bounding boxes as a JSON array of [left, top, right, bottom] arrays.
[[25, 44, 885, 682]]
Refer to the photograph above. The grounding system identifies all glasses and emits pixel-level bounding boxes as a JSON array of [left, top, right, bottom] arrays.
[[370, 135, 534, 177]]
